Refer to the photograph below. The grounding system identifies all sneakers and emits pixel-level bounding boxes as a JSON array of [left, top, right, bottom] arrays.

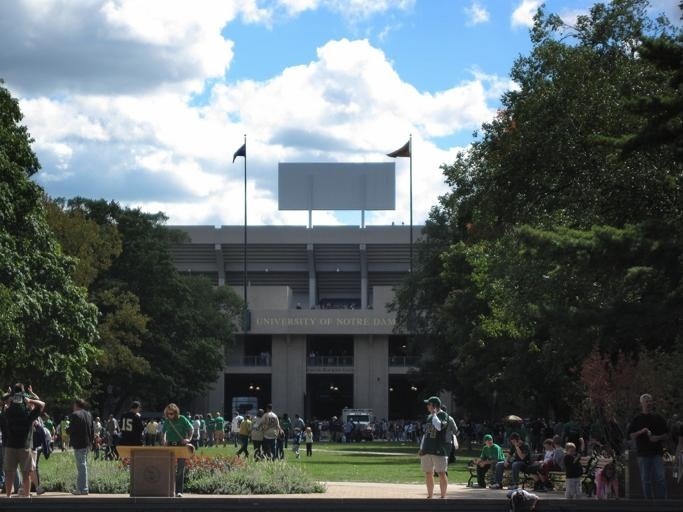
[[36, 487, 45, 494], [72, 490, 87, 495]]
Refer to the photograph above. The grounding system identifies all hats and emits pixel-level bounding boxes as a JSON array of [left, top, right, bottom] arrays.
[[423, 396, 441, 405], [482, 434, 492, 442]]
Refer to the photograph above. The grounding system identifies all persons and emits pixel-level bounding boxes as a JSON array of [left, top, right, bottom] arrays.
[[472, 432, 620, 499], [311, 416, 563, 453], [94, 400, 313, 461], [57, 415, 73, 451], [626, 394, 668, 499], [2, 383, 57, 498], [419, 396, 458, 498], [65, 399, 94, 495], [159, 403, 194, 496]]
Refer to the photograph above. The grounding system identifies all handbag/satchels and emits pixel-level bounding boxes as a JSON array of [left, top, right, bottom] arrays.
[[184, 443, 195, 457]]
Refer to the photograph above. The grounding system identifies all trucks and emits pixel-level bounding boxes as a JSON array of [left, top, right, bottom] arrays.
[[341, 407, 377, 441]]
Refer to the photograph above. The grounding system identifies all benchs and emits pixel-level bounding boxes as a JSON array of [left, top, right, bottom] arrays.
[[466, 450, 546, 489], [524, 455, 594, 497]]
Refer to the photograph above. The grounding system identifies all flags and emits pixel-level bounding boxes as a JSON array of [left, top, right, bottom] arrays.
[[385, 141, 410, 159], [232, 142, 244, 163]]
